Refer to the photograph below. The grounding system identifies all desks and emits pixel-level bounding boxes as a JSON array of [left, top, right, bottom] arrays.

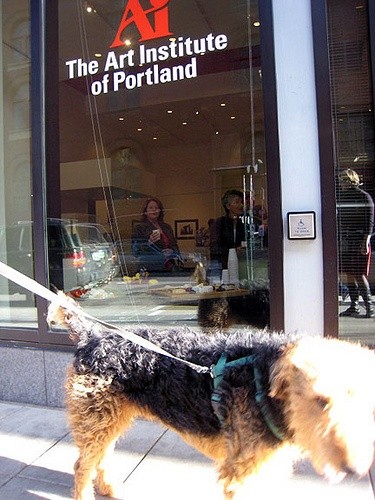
[[151, 285, 253, 304]]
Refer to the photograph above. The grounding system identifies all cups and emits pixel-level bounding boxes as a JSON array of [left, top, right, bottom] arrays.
[[222, 248, 239, 286], [153, 230, 160, 240]]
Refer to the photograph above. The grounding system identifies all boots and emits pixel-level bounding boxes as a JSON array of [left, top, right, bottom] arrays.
[[360, 288, 375, 317], [340, 285, 361, 316]]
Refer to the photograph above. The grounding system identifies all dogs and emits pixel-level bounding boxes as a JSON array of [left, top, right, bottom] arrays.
[[47, 291, 375, 500], [197, 280, 272, 332]]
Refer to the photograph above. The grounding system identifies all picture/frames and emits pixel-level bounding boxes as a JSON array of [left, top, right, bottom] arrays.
[[174, 219, 199, 240]]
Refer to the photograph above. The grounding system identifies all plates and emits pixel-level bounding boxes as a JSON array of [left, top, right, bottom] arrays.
[[77, 294, 117, 301]]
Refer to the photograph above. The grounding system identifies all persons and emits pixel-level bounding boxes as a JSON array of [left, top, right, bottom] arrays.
[[208, 191, 252, 279], [339, 169, 375, 318], [132, 199, 184, 273], [254, 205, 268, 248], [204, 219, 215, 246]]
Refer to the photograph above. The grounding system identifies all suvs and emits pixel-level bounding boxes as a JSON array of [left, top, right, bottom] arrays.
[[114, 239, 185, 276], [5, 218, 120, 301]]
